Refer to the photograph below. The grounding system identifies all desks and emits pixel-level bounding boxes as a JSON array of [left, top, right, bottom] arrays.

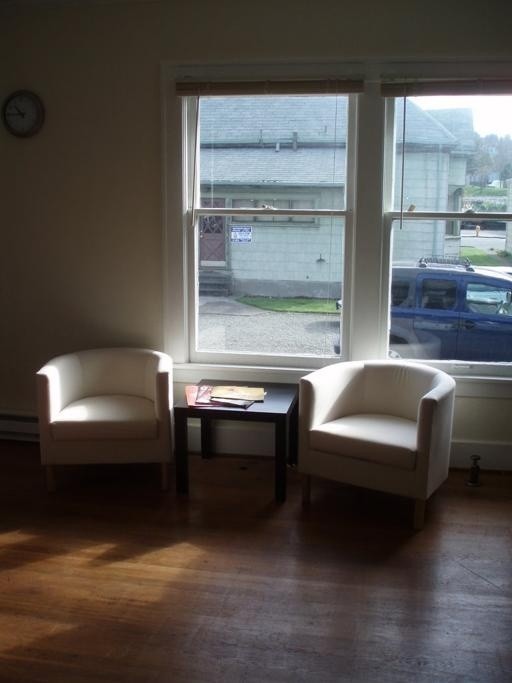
[[172, 377, 299, 505]]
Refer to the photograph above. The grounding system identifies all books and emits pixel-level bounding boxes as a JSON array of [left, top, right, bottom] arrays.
[[184, 384, 265, 407]]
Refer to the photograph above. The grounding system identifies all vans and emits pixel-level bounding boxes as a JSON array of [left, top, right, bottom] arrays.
[[335, 258, 512, 363]]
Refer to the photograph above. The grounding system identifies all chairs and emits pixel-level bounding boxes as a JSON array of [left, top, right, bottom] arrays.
[[36, 345, 175, 495], [294, 357, 458, 531]]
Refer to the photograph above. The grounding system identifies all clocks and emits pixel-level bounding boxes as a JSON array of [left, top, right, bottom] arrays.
[[5, 91, 46, 141]]
